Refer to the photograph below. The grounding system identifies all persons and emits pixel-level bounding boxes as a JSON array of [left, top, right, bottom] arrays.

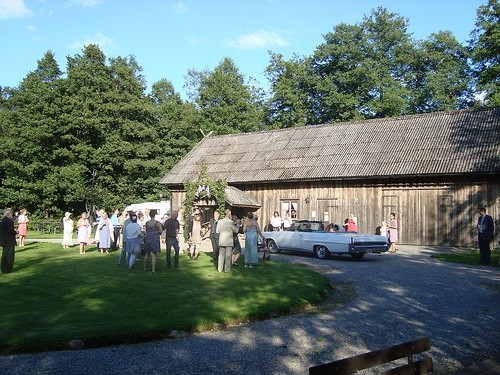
[[283, 213, 292, 230], [160, 211, 180, 268], [18, 209, 29, 247], [126, 214, 143, 270], [375, 213, 398, 253], [216, 210, 240, 272], [137, 212, 144, 231], [243, 212, 265, 268], [77, 212, 90, 254], [270, 211, 282, 231], [343, 212, 357, 232], [93, 209, 119, 254], [209, 210, 220, 259], [232, 215, 244, 266], [62, 212, 74, 248], [143, 210, 163, 273], [0, 209, 19, 273], [477, 208, 493, 266], [189, 208, 202, 260], [119, 211, 134, 264]]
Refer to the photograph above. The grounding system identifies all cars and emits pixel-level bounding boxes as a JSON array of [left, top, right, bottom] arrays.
[[201, 225, 265, 251], [261, 219, 392, 259]]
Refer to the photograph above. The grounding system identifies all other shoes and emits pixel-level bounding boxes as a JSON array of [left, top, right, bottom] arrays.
[[249, 266, 254, 268], [245, 265, 248, 267]]
